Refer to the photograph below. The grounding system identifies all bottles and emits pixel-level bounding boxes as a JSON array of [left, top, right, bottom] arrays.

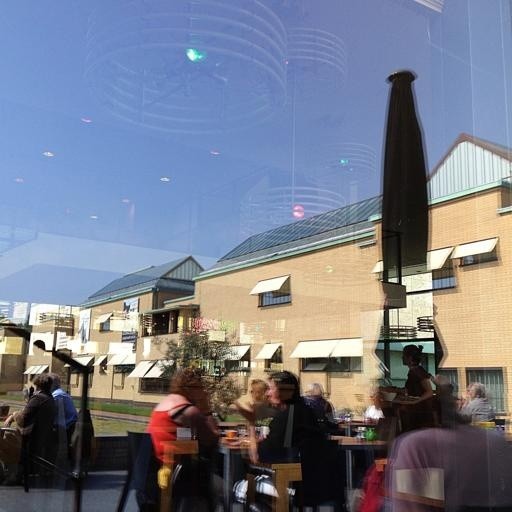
[[357, 426, 375, 441], [344, 411, 351, 422]]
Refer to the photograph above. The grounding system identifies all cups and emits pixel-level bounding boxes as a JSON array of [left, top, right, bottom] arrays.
[[223, 429, 239, 439]]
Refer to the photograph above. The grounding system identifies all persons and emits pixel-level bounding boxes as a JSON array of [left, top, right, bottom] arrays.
[[2, 374, 56, 485], [23, 383, 30, 404], [46, 372, 78, 476], [145, 344, 511, 509]]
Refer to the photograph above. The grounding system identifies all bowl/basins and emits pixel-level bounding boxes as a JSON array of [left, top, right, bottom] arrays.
[[380, 392, 398, 401]]
